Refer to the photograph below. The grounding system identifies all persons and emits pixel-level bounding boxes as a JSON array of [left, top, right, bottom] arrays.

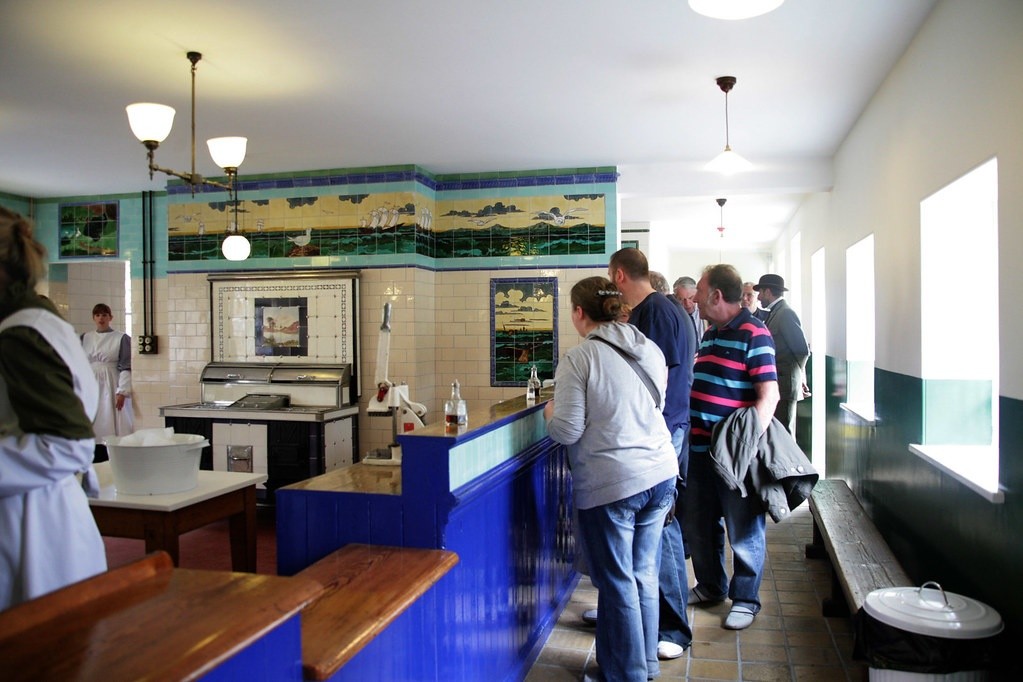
[[609, 247, 810, 441], [80, 303, 132, 444], [544, 276, 679, 680], [0, 201, 107, 608], [580, 249, 693, 657], [687, 264, 779, 629]]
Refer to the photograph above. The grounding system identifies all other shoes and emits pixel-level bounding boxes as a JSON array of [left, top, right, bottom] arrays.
[[583, 609, 599, 623], [583, 672, 600, 682], [656, 641, 684, 658]]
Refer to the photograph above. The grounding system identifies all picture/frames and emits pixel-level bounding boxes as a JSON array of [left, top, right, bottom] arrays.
[[489, 277, 559, 386]]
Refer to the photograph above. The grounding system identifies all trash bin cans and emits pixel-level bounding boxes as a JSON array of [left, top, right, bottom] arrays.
[[862, 581, 1005, 682]]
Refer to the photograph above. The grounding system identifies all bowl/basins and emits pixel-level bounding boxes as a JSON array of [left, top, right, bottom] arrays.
[[101, 433, 211, 497]]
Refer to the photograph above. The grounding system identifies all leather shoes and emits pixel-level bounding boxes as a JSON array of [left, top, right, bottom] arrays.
[[724, 606, 756, 628], [685, 588, 711, 604]]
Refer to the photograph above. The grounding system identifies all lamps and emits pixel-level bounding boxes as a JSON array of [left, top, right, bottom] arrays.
[[125, 52, 249, 198], [704, 76, 750, 174], [221, 170, 251, 261]]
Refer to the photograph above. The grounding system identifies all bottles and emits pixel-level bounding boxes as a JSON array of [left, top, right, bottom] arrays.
[[527, 382, 535, 400], [445, 382, 467, 426], [528, 367, 541, 396]]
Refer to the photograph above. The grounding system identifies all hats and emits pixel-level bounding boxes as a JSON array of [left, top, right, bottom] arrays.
[[753, 274, 788, 291]]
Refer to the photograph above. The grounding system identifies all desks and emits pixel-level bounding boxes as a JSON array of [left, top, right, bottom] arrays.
[[87, 471, 267, 573]]
